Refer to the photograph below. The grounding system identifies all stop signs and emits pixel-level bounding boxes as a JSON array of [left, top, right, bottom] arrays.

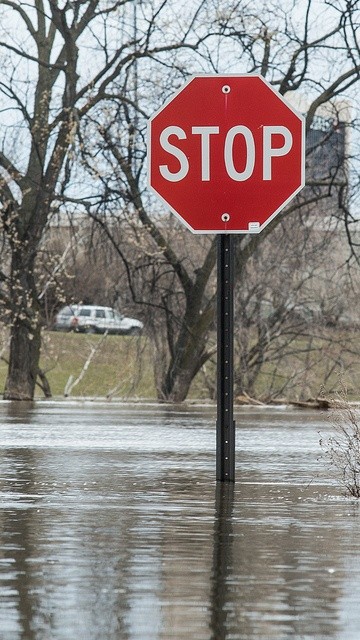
[[148, 74, 305, 234]]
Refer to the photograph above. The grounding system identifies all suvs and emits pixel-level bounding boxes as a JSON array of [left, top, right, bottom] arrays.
[[56, 305, 143, 335]]
